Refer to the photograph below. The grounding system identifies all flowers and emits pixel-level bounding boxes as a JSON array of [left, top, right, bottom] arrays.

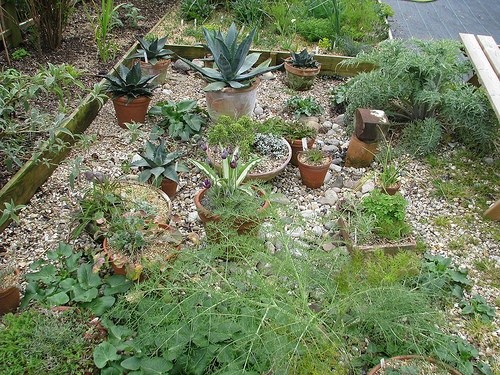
[[94, 209, 164, 253], [187, 141, 270, 198], [67, 172, 158, 238]]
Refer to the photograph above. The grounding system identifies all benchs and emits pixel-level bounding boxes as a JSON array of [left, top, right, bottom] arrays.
[[459, 32, 500, 222]]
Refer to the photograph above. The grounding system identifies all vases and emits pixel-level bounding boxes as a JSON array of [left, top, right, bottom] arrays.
[[194, 183, 270, 262], [80, 179, 174, 239], [103, 223, 182, 281]]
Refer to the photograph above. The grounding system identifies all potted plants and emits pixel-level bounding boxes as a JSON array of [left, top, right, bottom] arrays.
[[282, 119, 318, 166], [359, 123, 421, 195], [125, 136, 188, 196], [280, 48, 323, 91], [128, 33, 176, 86], [205, 115, 292, 183], [0, 249, 19, 316], [176, 22, 286, 125], [296, 140, 331, 189], [95, 58, 162, 130], [338, 189, 417, 259]]
